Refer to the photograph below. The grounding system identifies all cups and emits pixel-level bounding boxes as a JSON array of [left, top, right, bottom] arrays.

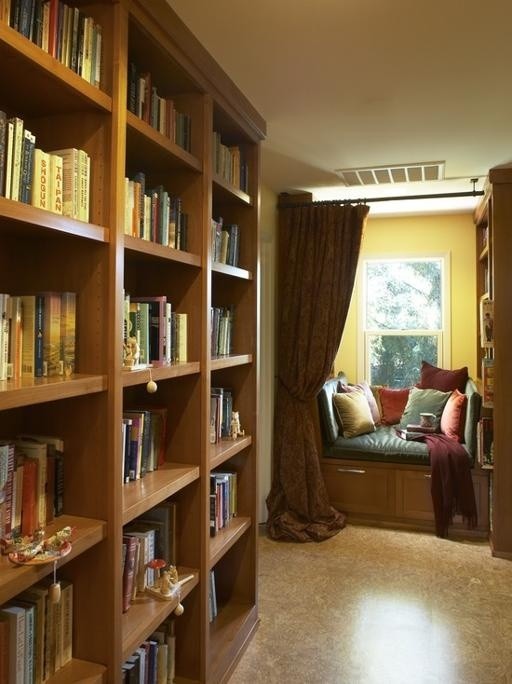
[[420, 413, 436, 427]]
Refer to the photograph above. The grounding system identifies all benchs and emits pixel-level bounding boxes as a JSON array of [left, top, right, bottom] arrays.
[[314, 368, 482, 468]]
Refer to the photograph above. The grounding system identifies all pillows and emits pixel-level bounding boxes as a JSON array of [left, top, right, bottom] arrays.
[[330, 358, 469, 445]]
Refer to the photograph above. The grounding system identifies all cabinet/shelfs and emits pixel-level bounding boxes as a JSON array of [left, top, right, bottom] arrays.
[[317, 461, 481, 528], [471, 183, 512, 560], [1, 0, 269, 684]]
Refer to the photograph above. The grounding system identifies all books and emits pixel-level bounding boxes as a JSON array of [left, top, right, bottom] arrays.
[[396, 424, 440, 441], [476, 300, 494, 471], [121, 65, 189, 684], [1, 0, 101, 682], [209, 130, 240, 624]]
